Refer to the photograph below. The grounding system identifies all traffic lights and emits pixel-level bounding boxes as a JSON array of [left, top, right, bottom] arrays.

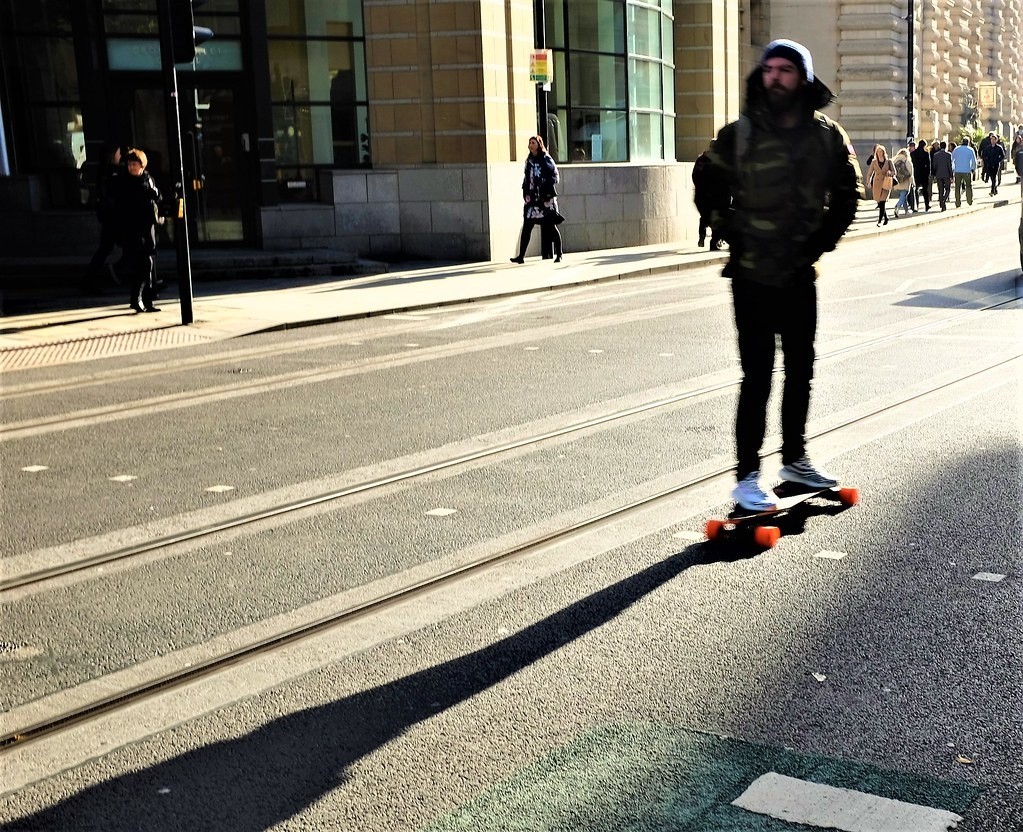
[[155, 0, 215, 65]]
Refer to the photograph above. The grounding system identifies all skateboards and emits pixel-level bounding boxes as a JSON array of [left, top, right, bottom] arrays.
[[706, 478, 859, 547]]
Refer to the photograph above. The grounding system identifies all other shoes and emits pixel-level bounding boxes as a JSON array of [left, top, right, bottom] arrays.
[[698, 239, 704, 248], [710, 245, 721, 251]]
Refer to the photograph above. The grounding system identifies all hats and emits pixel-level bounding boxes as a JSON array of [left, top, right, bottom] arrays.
[[765, 39, 815, 87]]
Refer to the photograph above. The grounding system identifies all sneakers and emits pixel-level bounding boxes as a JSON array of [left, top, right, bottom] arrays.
[[780, 453, 839, 490], [730, 477, 777, 512]]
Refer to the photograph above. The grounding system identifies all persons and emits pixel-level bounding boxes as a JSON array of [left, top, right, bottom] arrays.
[[709, 39, 862, 515], [510, 136, 565, 264], [692, 150, 722, 251], [866, 124, 1023, 228], [112, 148, 165, 314]]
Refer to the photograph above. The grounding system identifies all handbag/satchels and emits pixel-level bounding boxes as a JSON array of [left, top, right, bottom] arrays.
[[883, 177, 893, 190]]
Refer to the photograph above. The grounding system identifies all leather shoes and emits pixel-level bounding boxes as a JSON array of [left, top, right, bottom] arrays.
[[511, 256, 524, 263], [146, 303, 161, 311], [128, 300, 146, 312], [554, 253, 564, 263]]
